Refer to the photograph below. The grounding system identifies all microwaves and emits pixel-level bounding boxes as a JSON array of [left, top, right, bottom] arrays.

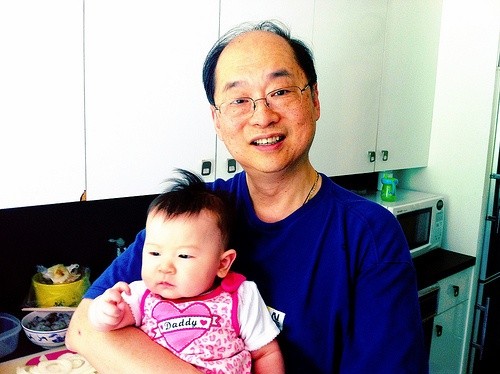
[[359, 188, 444, 258]]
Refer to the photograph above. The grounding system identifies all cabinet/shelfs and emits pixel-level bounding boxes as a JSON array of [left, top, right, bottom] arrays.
[[429, 265, 473, 374], [0, 1, 83, 209], [84, 1, 308, 200], [308, 0, 442, 178]]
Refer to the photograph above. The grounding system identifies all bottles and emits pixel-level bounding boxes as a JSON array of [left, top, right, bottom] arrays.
[[109, 238, 126, 257], [381, 175, 398, 202], [377, 171, 393, 191]]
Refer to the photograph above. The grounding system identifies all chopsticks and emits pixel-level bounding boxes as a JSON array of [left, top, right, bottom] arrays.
[[22, 307, 77, 311]]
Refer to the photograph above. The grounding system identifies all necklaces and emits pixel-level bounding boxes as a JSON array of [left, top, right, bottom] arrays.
[[302, 170, 319, 206]]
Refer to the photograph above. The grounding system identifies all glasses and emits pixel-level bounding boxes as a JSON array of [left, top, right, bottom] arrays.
[[213, 82, 311, 121]]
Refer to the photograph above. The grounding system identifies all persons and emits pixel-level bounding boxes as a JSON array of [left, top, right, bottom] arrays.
[[65, 20, 429, 374], [88, 167, 286, 374]]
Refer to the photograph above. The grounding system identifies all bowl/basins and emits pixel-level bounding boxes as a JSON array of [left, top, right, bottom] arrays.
[[0, 312, 22, 358], [21, 306, 74, 349], [32, 267, 91, 308]]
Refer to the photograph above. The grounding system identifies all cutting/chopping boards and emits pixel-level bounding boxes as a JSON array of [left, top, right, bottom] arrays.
[[0, 346, 99, 374]]
